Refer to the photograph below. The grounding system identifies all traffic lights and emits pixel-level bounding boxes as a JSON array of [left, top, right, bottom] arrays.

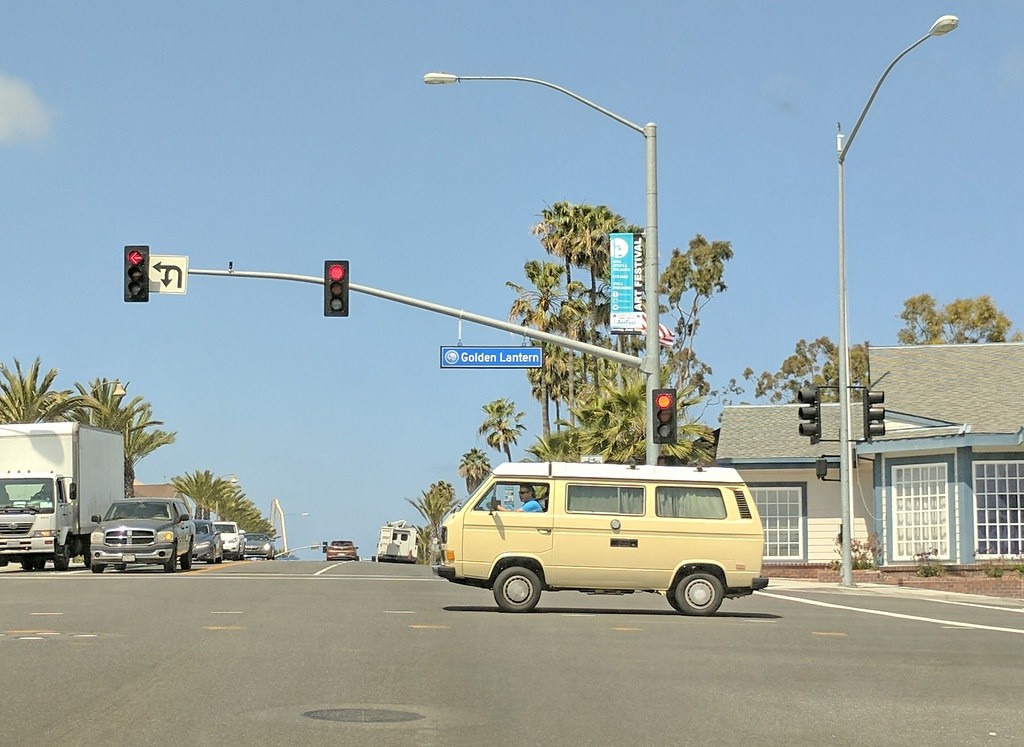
[[653, 388, 679, 444], [863, 388, 885, 440], [798, 386, 822, 444], [324, 260, 351, 319], [123, 245, 150, 303]]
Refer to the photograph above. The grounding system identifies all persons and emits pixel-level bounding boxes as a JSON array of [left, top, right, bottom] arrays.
[[494, 484, 543, 512], [32, 484, 52, 500]]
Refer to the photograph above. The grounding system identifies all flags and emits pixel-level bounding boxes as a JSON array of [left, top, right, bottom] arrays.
[[642, 313, 675, 346]]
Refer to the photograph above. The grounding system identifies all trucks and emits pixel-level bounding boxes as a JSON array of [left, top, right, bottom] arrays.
[[0, 422, 125, 571], [377, 520, 418, 564]]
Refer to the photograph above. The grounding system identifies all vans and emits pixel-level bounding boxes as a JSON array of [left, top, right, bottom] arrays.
[[431, 461, 769, 617]]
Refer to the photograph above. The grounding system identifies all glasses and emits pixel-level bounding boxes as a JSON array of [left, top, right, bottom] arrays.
[[519, 491, 528, 494]]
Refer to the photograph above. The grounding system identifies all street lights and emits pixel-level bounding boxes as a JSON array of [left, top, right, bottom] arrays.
[[422, 72, 659, 466], [835, 15, 960, 588]]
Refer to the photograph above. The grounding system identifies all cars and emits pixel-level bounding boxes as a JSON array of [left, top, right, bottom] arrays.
[[244, 533, 275, 560], [327, 540, 359, 561], [193, 520, 223, 564]]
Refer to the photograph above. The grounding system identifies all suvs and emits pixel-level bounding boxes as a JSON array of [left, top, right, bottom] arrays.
[[212, 522, 246, 561], [89, 498, 196, 573]]
